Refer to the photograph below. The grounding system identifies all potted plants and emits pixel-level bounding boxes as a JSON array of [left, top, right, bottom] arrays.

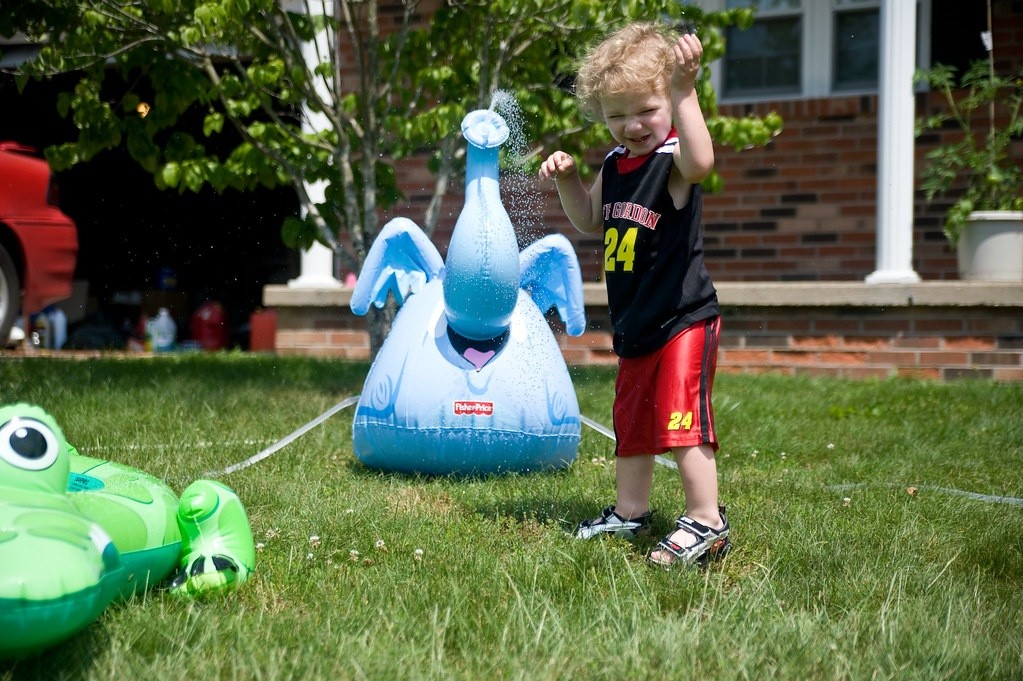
[[913, 61, 1023, 282]]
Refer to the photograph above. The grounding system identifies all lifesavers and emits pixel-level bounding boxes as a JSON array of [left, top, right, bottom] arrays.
[[0, 401, 257, 663]]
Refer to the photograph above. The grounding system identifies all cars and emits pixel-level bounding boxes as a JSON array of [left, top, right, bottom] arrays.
[[0, 141, 81, 353]]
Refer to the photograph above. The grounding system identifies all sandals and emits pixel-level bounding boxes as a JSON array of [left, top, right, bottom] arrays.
[[648, 506, 730, 565], [568, 506, 660, 541]]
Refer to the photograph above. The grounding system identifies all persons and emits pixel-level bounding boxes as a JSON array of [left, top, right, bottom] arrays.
[[539, 25, 732, 573]]
